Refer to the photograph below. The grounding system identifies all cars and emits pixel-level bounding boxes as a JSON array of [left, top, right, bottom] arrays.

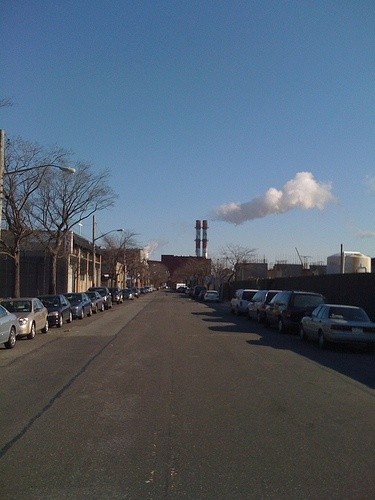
[[37, 294, 73, 328], [299, 303, 375, 350], [89, 287, 153, 310], [178, 287, 220, 303], [0, 298, 49, 340], [66, 293, 92, 320], [0, 305, 20, 349], [83, 291, 105, 314]]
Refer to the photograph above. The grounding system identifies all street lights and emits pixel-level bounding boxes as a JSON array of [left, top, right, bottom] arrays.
[[92, 214, 123, 286], [0, 128, 76, 223]]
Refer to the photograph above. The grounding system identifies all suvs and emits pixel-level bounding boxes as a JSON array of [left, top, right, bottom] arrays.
[[248, 290, 284, 324], [264, 290, 328, 335], [230, 289, 260, 316]]
